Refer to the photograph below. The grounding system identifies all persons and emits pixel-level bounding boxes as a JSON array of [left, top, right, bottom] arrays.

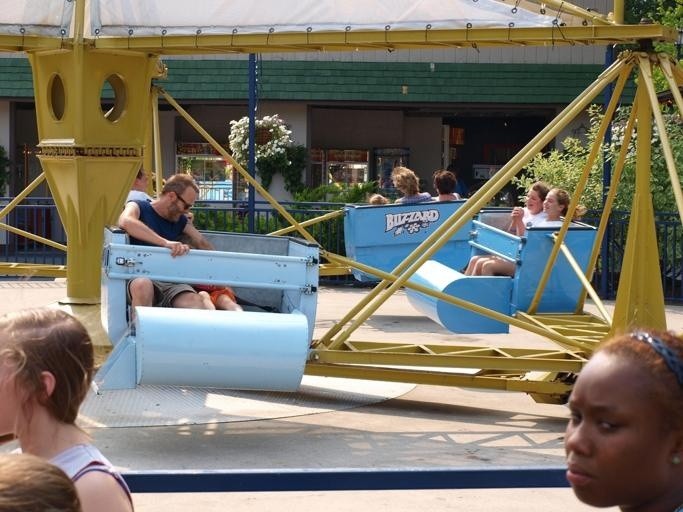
[[179, 234, 245, 311], [113, 172, 215, 327], [0, 445, 84, 510], [470, 187, 587, 277], [390, 165, 432, 205], [366, 194, 389, 206], [429, 169, 461, 202], [0, 306, 139, 512], [463, 181, 549, 275], [560, 324, 682, 511], [125, 166, 158, 203]]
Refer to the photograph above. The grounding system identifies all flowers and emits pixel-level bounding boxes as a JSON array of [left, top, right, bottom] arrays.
[[224, 112, 294, 174]]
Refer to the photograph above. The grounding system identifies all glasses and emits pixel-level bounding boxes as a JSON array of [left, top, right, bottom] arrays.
[[176, 194, 192, 211]]
